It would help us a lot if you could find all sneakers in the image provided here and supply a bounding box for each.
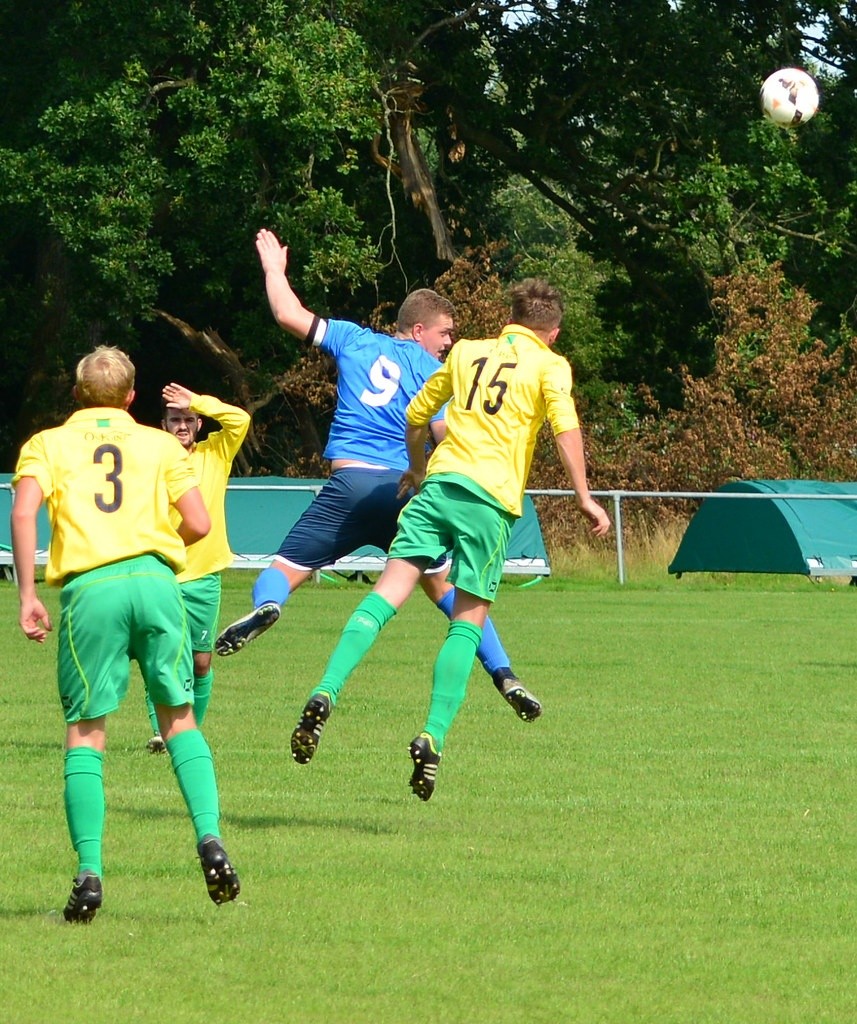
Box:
[195,837,241,905]
[146,732,170,759]
[290,692,333,764]
[500,678,544,723]
[407,733,441,801]
[214,602,281,656]
[63,867,102,925]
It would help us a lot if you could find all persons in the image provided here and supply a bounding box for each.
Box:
[145,383,250,754]
[290,276,610,802]
[10,345,241,923]
[213,228,542,723]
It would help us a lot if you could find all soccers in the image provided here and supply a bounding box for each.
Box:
[758,67,820,129]
